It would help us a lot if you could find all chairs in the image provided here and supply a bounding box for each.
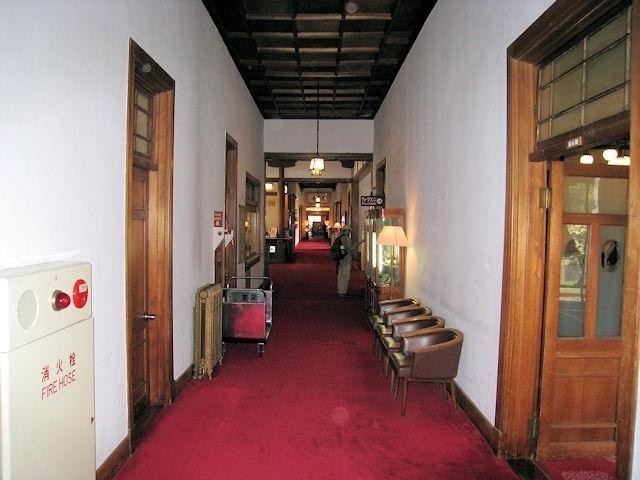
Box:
[369,298,463,417]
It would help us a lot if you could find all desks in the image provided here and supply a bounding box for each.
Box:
[264,235,283,264]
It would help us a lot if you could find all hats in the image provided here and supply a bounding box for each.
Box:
[343,226,352,231]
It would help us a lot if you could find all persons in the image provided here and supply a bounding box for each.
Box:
[334,224,355,298]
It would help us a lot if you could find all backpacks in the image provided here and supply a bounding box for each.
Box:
[332,234,347,261]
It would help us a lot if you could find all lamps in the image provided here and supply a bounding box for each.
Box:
[603,148,618,162]
[377,225,409,300]
[303,221,310,240]
[315,188,321,203]
[580,149,594,165]
[325,221,342,236]
[309,80,325,176]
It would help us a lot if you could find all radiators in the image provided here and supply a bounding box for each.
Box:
[193,284,223,380]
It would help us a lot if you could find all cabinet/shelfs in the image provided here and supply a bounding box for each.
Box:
[364,208,405,317]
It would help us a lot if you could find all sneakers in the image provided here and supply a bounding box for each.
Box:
[337,292,352,298]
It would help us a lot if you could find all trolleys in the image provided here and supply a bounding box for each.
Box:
[221,276,273,356]
